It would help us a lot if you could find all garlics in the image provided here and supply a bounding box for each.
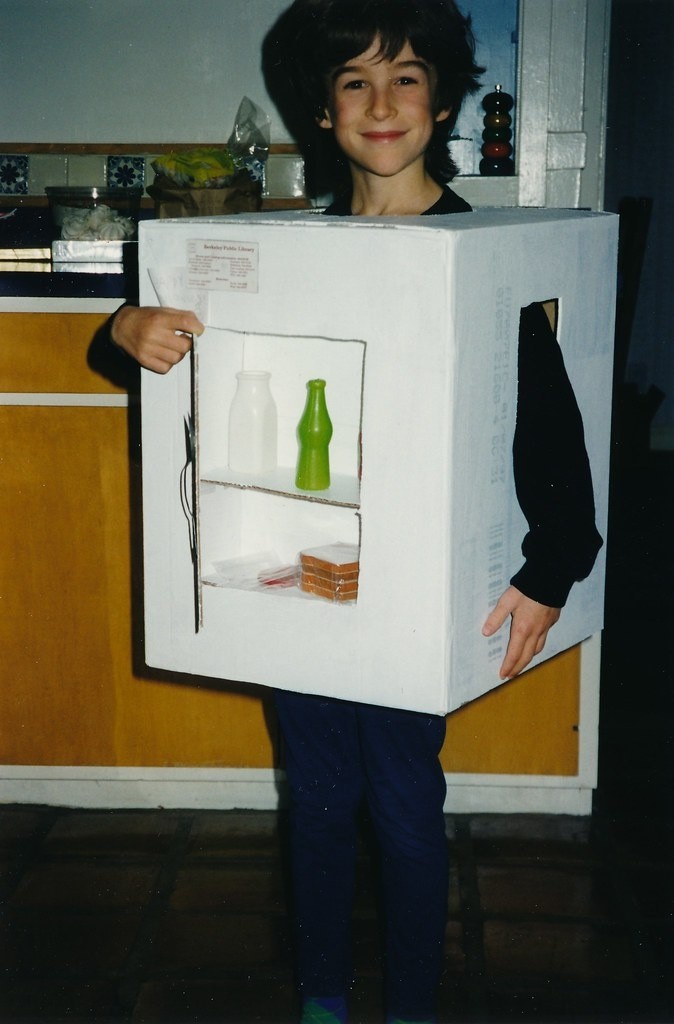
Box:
[61,206,135,241]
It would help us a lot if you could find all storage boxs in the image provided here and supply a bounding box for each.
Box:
[136,209,621,716]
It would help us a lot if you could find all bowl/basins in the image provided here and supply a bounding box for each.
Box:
[45,185,146,241]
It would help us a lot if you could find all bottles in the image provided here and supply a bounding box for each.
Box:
[227,372,277,479]
[297,379,335,491]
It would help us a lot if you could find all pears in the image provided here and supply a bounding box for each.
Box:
[156,149,235,187]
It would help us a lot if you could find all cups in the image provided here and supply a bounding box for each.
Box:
[448,139,477,176]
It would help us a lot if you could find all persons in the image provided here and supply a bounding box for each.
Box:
[110,0,603,1024]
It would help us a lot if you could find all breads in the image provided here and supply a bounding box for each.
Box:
[300,543,360,601]
